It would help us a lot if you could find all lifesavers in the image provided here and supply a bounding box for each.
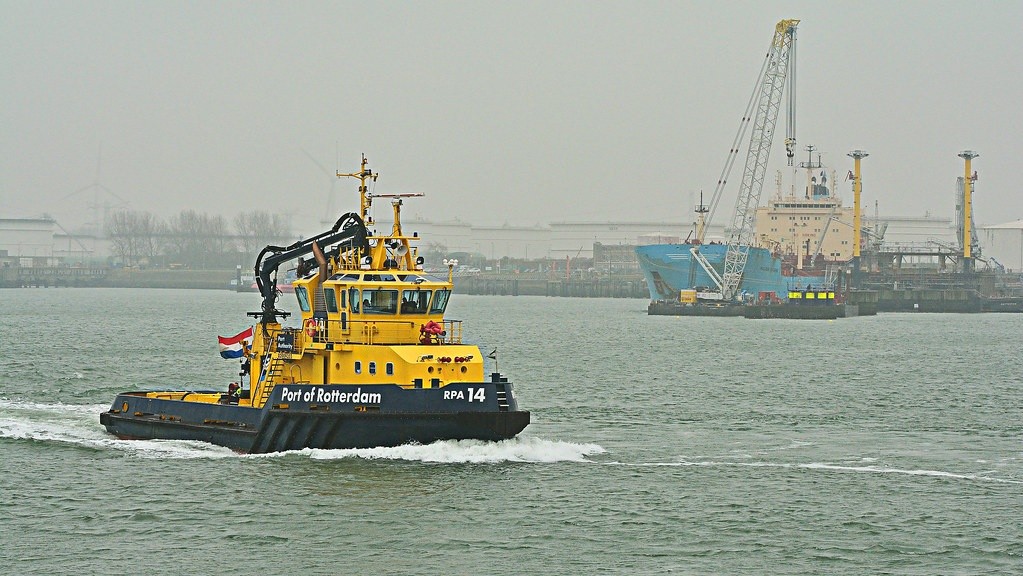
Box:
[307,319,316,337]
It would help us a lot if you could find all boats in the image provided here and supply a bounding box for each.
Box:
[634,167,870,300]
[98,151,534,452]
[229,264,315,295]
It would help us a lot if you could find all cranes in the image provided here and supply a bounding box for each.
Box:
[683,19,798,304]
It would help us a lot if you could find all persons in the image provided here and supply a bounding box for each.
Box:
[228,382,241,397]
[363,299,371,307]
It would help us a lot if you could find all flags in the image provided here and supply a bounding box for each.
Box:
[486,350,495,359]
[218,326,254,359]
